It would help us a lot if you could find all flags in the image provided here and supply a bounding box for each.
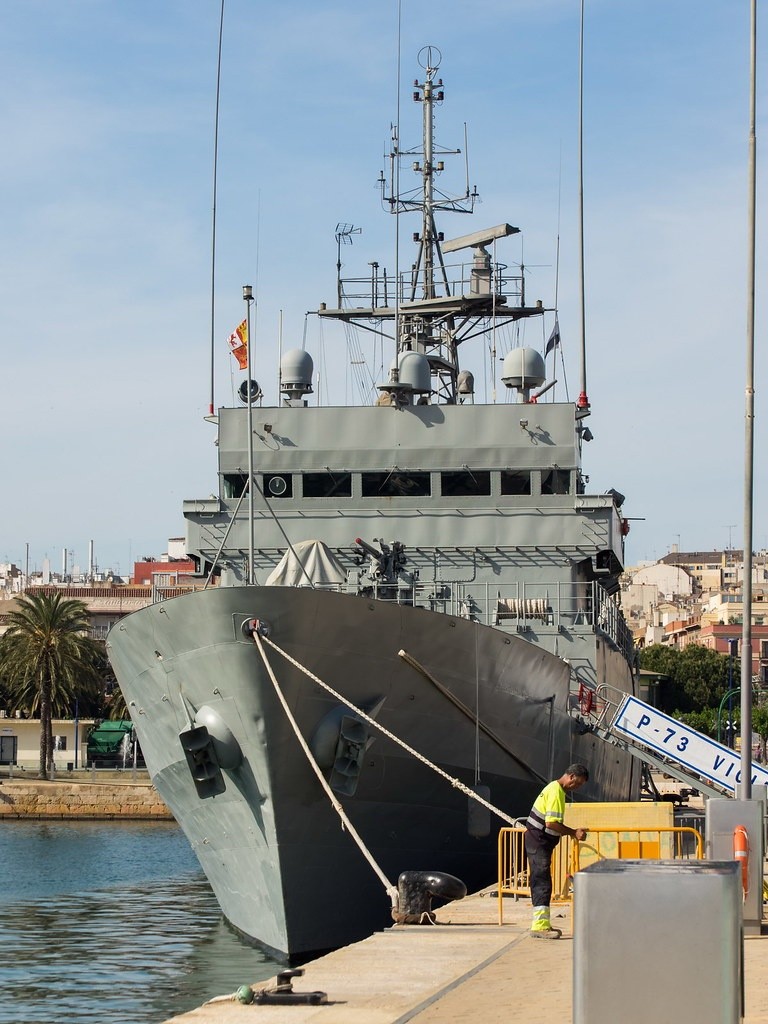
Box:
[226,318,248,369]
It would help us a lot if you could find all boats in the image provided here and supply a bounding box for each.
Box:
[106,0,656,965]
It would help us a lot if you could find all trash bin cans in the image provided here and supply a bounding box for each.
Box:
[66,763,73,770]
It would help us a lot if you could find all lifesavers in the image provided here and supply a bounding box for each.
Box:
[732,821,750,902]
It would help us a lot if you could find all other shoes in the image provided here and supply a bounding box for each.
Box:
[531,928,562,939]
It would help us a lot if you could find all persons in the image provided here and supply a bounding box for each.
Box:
[524,764,589,939]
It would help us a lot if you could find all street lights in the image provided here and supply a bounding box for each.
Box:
[725,636,739,749]
